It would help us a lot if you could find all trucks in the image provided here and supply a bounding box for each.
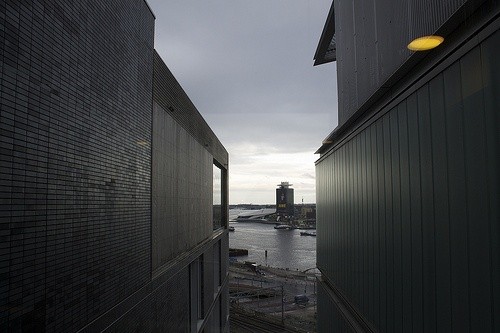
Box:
[245,261,261,272]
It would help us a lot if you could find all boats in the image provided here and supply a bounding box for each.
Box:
[300,231,316,237]
[229,225,235,231]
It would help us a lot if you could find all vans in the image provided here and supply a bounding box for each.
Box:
[295,294,309,304]
